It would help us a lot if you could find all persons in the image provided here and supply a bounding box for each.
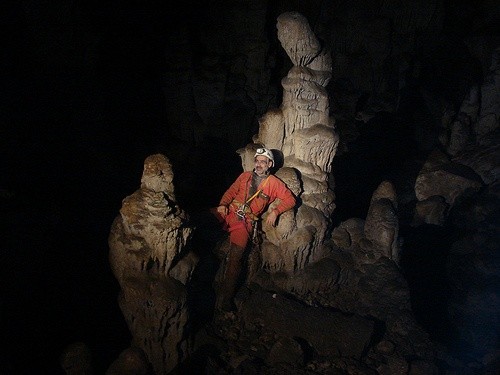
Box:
[188,148,296,314]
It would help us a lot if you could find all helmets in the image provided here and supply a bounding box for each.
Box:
[254,148,274,168]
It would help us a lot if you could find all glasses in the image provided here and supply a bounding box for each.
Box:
[254,160,268,164]
